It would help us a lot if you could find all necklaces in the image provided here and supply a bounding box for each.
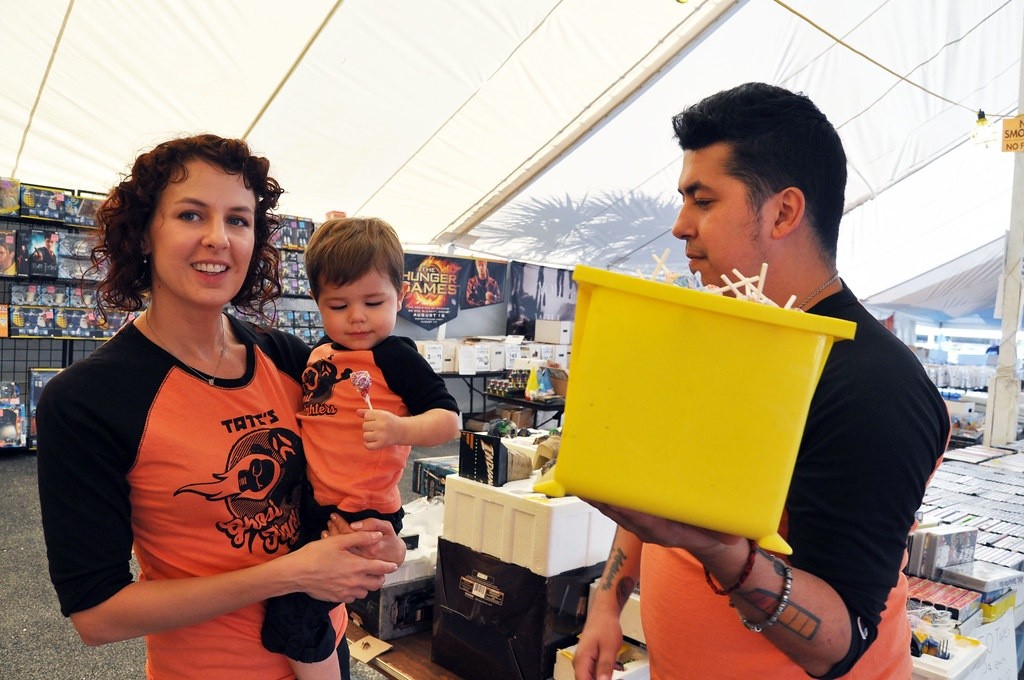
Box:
[797,272,838,311]
[146,308,225,386]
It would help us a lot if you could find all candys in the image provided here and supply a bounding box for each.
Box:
[349,370,373,409]
[633,248,807,313]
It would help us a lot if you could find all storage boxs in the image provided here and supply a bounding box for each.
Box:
[533,265,858,555]
[443,473,617,577]
[412,456,459,497]
[431,535,606,680]
[414,319,573,375]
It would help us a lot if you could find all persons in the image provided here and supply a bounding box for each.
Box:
[466,260,501,306]
[0,240,16,276]
[35,135,407,680]
[261,218,460,679]
[573,82,955,680]
[31,234,57,274]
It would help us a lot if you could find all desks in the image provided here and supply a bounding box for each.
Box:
[436,369,513,412]
[482,393,566,429]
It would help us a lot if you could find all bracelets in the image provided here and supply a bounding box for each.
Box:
[742,558,790,632]
[703,542,755,595]
